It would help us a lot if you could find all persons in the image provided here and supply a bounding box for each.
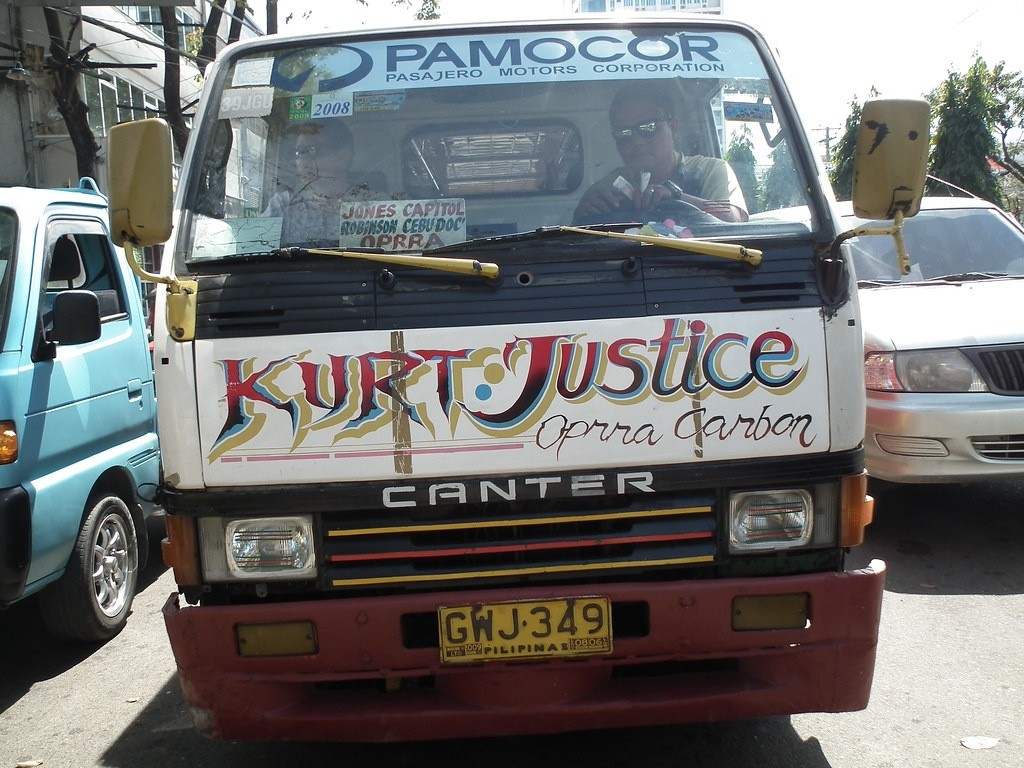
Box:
[259,117,392,243]
[571,87,750,227]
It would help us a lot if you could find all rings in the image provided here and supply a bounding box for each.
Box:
[649,188,655,192]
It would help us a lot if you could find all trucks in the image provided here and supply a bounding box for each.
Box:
[102,4,939,752]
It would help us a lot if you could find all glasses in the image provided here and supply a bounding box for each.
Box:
[613,115,673,146]
[294,143,342,161]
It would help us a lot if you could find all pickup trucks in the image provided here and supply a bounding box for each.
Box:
[0,179,178,644]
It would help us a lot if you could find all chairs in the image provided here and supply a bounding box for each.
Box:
[47,238,81,288]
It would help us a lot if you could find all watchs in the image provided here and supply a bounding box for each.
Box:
[662,180,683,200]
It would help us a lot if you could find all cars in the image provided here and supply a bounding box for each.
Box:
[738,198,1023,496]
